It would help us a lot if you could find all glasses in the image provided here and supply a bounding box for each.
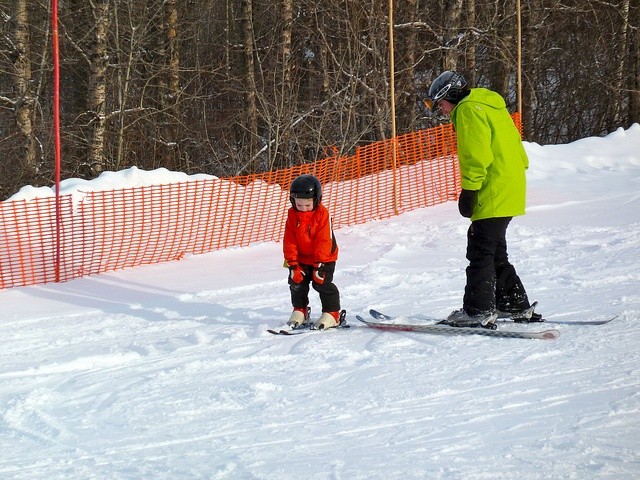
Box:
[422,84,452,113]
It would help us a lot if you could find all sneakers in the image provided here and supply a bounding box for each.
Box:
[494,307,530,318]
[315,308,341,329]
[287,308,308,328]
[448,307,497,325]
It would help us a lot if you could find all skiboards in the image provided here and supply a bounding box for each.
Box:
[356,310,619,339]
[267,311,350,335]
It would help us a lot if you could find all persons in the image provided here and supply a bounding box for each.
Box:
[282,174,341,330]
[427,70,531,324]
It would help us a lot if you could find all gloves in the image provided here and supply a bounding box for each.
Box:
[458,189,479,218]
[287,261,306,284]
[312,262,326,284]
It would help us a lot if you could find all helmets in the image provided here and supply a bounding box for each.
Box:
[424,70,468,113]
[290,174,322,210]
